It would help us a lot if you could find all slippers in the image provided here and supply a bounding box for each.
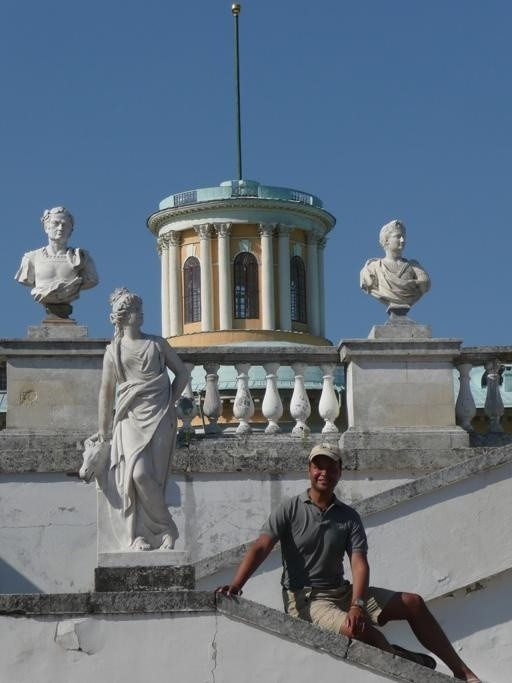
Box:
[391,644,437,670]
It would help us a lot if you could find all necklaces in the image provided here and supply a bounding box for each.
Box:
[309,495,335,511]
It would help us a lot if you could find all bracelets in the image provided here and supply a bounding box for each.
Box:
[350,598,369,610]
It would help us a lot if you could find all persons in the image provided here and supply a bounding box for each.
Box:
[357,218,431,310]
[94,283,188,553]
[14,206,99,306]
[216,443,480,683]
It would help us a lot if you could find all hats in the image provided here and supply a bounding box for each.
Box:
[307,442,344,463]
